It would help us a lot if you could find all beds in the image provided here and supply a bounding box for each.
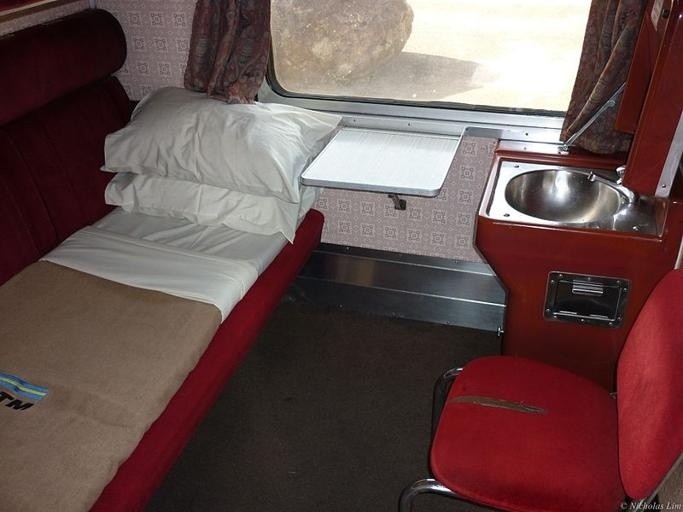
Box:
[0,8,323,512]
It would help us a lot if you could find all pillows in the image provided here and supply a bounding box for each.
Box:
[100,87,344,245]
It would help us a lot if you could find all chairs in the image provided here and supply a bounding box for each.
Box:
[398,269,683,512]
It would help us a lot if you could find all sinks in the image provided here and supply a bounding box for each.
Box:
[505,170,634,224]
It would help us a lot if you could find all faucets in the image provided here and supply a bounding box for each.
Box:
[616,165,626,185]
